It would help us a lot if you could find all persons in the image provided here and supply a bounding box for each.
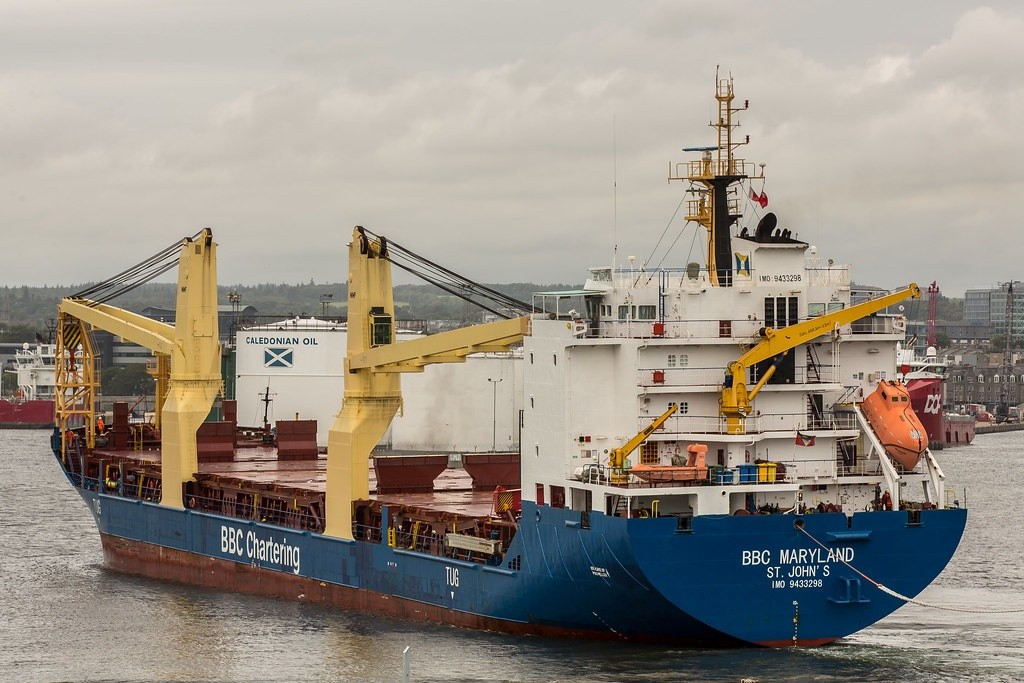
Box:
[875,486,893,511]
[66,428,75,448]
[97,417,105,434]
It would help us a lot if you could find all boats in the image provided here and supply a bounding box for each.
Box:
[0,332,90,428]
[899,372,976,450]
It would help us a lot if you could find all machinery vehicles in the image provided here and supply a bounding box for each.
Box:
[996,281,1021,425]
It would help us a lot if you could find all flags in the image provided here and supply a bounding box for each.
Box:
[749,186,768,209]
[795,431,816,446]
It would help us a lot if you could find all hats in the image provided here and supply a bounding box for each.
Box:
[99,417,102,419]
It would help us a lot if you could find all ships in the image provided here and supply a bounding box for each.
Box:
[49,66,969,652]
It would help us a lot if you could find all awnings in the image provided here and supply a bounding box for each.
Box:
[530,289,605,321]
